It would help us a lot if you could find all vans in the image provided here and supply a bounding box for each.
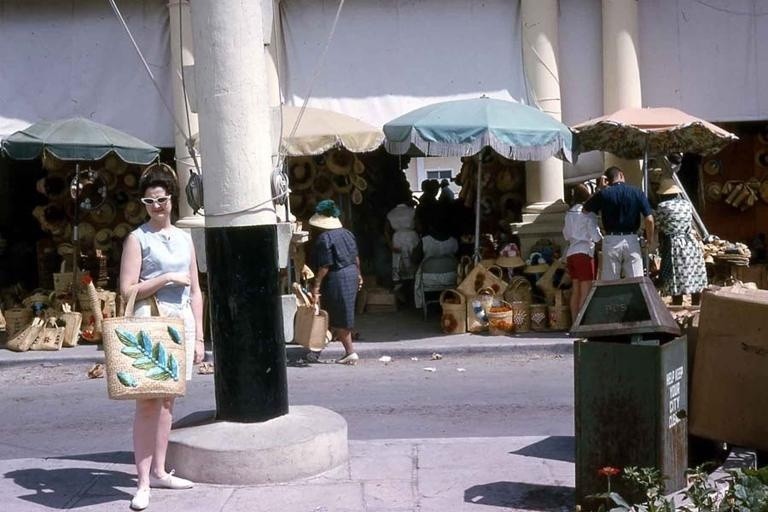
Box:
[336,351,359,365]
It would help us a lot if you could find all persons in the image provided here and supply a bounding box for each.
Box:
[423,179,440,207]
[581,165,655,281]
[654,178,710,306]
[309,199,363,364]
[117,165,206,510]
[418,180,429,204]
[439,179,453,203]
[410,214,459,311]
[561,184,601,326]
[383,195,422,284]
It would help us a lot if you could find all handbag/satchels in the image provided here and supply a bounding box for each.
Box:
[314,277,320,289]
[196,335,205,343]
[594,187,601,193]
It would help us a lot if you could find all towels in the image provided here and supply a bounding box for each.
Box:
[569,278,688,512]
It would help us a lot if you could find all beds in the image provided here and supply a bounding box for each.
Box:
[572,104,740,198]
[189,102,384,218]
[1,117,164,313]
[382,94,573,264]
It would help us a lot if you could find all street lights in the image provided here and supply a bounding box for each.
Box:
[294,296,328,352]
[439,265,569,335]
[1,259,119,353]
[100,282,190,401]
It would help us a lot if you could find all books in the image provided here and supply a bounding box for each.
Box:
[691,285,767,455]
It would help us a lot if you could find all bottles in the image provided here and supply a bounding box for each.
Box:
[494,243,550,275]
[307,199,344,229]
[285,149,357,219]
[32,156,147,259]
[455,146,525,222]
[655,177,684,195]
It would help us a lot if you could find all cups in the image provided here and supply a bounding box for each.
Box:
[141,195,173,205]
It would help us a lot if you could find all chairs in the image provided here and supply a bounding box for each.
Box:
[130,485,150,511]
[149,471,197,490]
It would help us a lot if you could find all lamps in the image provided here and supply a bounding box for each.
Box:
[419,252,463,320]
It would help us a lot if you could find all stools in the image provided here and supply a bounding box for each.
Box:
[604,230,639,236]
